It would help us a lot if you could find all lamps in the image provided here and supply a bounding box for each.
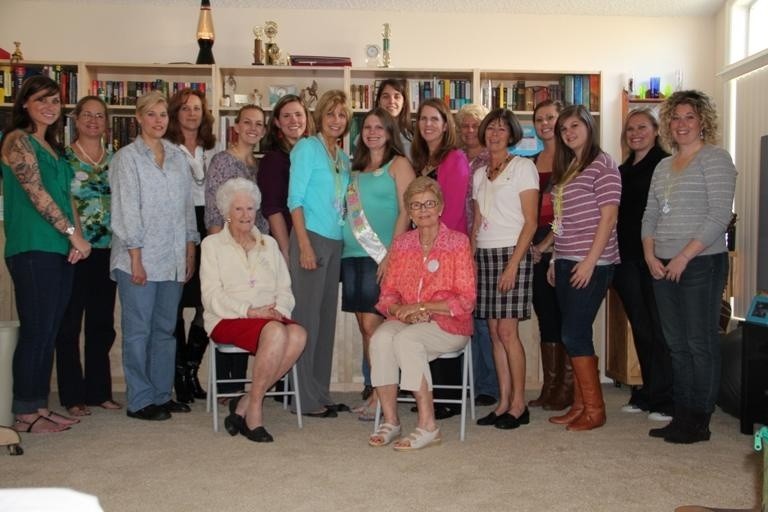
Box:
[196,0,217,63]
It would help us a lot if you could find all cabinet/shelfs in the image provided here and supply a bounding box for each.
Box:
[78,63,215,393]
[474,71,604,391]
[605,85,733,394]
[0,60,78,393]
[215,68,347,392]
[349,70,474,391]
[738,319,767,435]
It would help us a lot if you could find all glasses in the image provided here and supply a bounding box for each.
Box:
[77,110,106,122]
[410,199,437,210]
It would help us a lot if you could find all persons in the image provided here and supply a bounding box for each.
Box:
[1,64,207,162]
[2,75,575,433]
[198,174,307,443]
[613,106,679,424]
[637,87,739,443]
[544,103,624,431]
[368,173,480,450]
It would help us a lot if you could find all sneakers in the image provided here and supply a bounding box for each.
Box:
[622,403,649,413]
[663,424,710,443]
[648,413,674,420]
[362,385,372,400]
[397,387,407,403]
[648,422,677,437]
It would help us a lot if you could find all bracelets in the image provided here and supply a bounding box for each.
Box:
[418,302,426,309]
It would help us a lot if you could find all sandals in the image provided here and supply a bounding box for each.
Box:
[368,423,400,448]
[47,411,80,424]
[393,426,441,451]
[15,415,71,432]
[217,397,231,405]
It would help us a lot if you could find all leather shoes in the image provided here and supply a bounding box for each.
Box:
[324,403,350,412]
[240,420,273,443]
[411,406,418,412]
[495,406,530,430]
[158,399,191,413]
[434,407,461,419]
[477,410,510,425]
[291,407,337,419]
[126,404,172,421]
[224,395,245,437]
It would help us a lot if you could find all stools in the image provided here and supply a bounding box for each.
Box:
[205,338,304,430]
[373,336,476,444]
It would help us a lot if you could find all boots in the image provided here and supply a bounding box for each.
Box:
[186,323,209,400]
[528,342,562,407]
[549,367,585,425]
[542,343,574,411]
[174,318,196,404]
[566,356,607,431]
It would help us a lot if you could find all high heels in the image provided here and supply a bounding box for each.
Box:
[86,399,121,410]
[67,402,91,416]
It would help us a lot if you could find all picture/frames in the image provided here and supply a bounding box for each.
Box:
[744,295,768,324]
